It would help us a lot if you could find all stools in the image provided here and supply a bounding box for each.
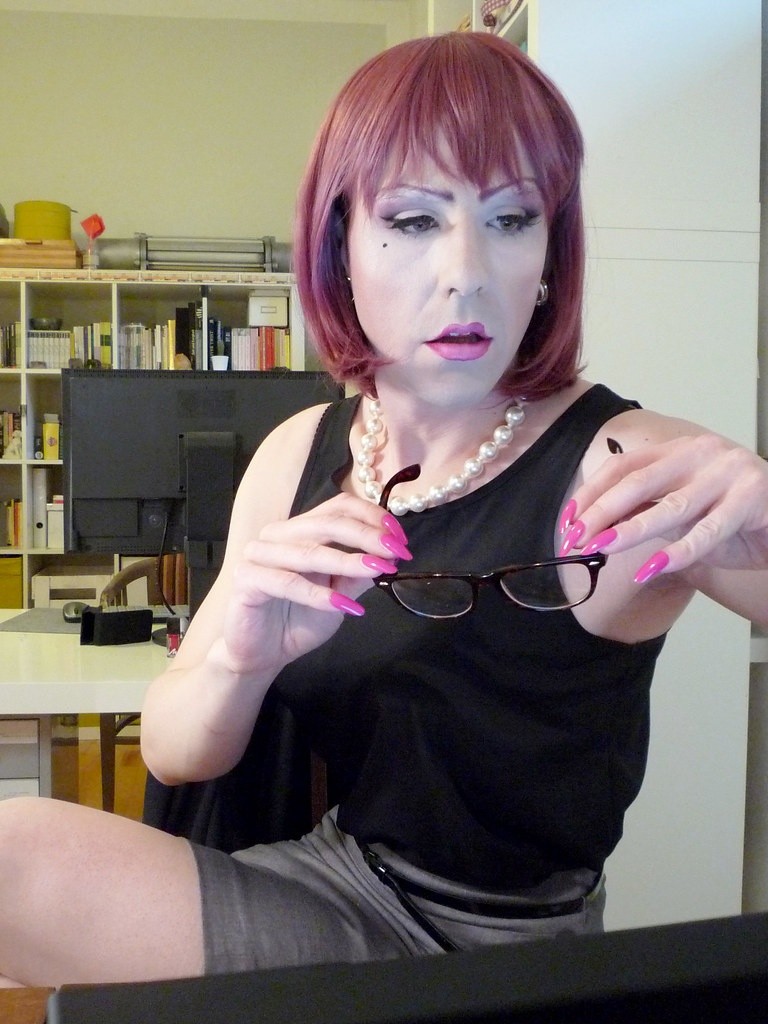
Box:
[32,565,115,606]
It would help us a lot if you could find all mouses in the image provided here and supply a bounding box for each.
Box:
[63,602,87,624]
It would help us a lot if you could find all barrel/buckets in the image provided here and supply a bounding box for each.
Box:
[14,200,77,239]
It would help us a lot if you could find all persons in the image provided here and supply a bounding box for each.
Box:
[0,34,768,989]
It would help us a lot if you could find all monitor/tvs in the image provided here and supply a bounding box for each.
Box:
[59,369,345,620]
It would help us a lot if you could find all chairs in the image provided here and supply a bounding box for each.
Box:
[102,556,187,816]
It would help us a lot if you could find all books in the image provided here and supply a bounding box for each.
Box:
[0,300,292,549]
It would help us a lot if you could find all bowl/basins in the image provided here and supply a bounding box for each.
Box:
[29,319,62,329]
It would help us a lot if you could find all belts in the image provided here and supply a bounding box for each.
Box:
[348,838,606,952]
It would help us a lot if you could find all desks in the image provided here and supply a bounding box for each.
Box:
[1,609,178,801]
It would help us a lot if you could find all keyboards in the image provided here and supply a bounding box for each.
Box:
[103,605,190,623]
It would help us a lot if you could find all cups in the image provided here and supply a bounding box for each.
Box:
[211,355,229,371]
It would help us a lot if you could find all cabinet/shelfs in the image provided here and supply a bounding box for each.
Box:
[428,0,760,933]
[0,268,307,744]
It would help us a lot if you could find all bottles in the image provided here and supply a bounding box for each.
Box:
[83,233,100,281]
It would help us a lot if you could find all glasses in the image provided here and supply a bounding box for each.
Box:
[376,436,626,621]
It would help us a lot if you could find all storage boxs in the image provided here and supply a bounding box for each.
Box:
[247,296,288,328]
[47,504,64,547]
[1,238,82,269]
[43,422,64,460]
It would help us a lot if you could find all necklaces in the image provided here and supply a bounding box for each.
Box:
[357,392,526,517]
